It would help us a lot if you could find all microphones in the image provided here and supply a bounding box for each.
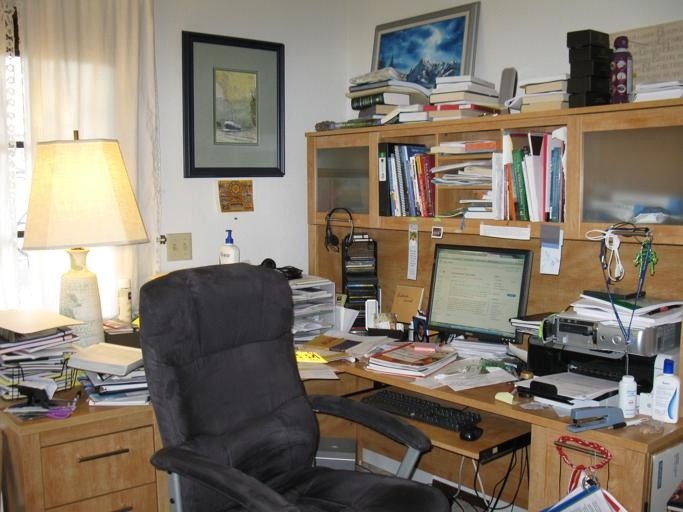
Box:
[345,247,351,262]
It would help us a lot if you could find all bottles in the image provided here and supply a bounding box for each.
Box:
[118,278,132,323]
[609,36,633,104]
[620,374,637,420]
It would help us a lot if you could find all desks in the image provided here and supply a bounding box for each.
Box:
[1,348,682,512]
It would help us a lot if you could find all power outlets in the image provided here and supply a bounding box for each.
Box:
[165,233,193,261]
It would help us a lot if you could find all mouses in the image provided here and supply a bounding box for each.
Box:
[460,426,483,441]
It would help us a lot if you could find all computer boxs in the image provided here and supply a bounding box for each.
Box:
[541,309,681,357]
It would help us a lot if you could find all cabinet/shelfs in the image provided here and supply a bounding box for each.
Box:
[304,100,682,347]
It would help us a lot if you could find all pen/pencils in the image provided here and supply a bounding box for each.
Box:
[608,418,642,430]
[70,390,81,411]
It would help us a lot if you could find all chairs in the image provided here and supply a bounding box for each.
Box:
[138,261,449,511]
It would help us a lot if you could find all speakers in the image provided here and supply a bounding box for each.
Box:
[365,299,378,331]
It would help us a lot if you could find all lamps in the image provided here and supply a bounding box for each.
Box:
[22,130,151,346]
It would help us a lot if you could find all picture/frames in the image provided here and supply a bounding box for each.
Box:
[181,30,287,178]
[369,1,479,91]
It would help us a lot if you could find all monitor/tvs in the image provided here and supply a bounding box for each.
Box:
[425,243,533,344]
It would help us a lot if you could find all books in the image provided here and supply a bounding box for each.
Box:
[375,126,569,226]
[633,80,682,102]
[288,276,458,386]
[0,304,152,429]
[505,73,570,113]
[508,289,683,337]
[326,66,508,130]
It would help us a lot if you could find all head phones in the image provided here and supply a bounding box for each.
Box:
[325,207,354,247]
[261,258,301,278]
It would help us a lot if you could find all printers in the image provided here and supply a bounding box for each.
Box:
[517,335,657,410]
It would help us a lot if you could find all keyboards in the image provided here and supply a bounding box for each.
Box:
[361,389,481,432]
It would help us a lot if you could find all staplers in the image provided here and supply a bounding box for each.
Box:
[566,406,624,433]
